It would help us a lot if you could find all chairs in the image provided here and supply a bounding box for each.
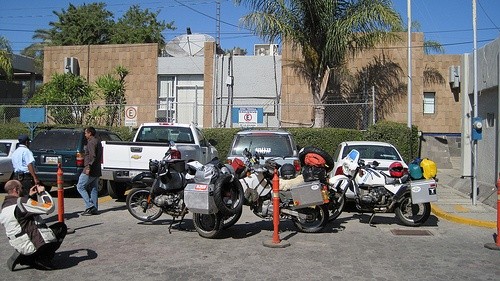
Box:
[142,133,157,142]
[176,132,189,142]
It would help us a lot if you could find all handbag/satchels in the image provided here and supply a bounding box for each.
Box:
[194,157,223,185]
[408,155,437,179]
[303,153,328,182]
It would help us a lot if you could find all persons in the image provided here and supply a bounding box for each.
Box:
[2,179,67,272]
[11,134,40,203]
[77,127,102,216]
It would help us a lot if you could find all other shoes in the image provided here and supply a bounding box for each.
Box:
[35,258,54,270]
[91,212,97,215]
[8,252,19,271]
[83,207,96,215]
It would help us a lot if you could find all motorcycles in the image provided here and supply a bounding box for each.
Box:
[219,140,337,234]
[125,140,244,238]
[315,148,439,227]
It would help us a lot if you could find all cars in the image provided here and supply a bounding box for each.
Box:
[326,140,409,213]
[0,139,29,193]
[25,126,124,197]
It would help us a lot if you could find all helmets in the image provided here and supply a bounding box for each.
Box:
[280,164,296,178]
[389,162,404,177]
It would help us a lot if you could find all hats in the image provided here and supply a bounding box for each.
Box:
[18,135,31,141]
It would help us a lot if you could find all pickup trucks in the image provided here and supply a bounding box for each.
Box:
[99,122,219,201]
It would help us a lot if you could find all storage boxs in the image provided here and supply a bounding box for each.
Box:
[411,179,437,205]
[290,181,326,206]
[183,183,219,215]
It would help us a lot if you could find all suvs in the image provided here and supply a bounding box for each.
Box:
[225,128,302,178]
